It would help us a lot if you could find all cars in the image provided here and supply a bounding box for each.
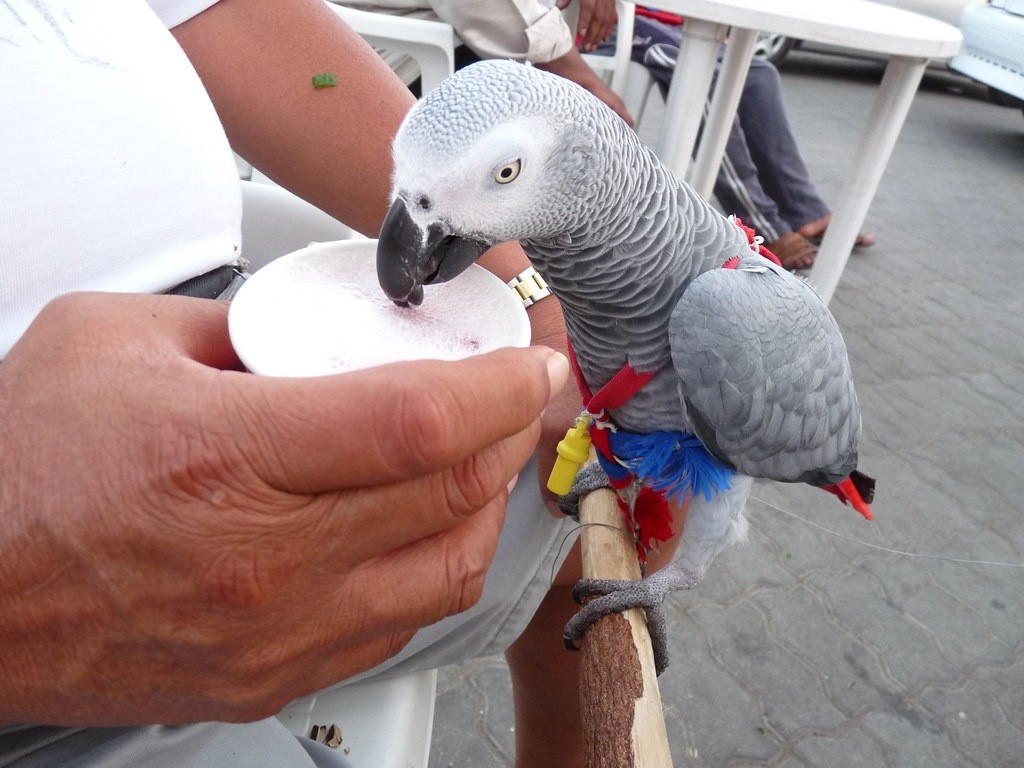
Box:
[717,0,993,95]
[953,0,1023,102]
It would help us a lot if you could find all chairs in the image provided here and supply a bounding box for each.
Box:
[251,0,454,185]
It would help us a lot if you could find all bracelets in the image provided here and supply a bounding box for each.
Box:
[507,266,554,308]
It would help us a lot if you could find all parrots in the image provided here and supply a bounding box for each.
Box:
[374,56,877,679]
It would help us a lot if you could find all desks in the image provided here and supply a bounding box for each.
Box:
[609,0,961,306]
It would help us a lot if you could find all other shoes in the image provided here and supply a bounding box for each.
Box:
[762,212,877,269]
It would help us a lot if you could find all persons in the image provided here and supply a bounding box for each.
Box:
[0,0,584,768]
[326,0,634,130]
[577,0,877,270]
